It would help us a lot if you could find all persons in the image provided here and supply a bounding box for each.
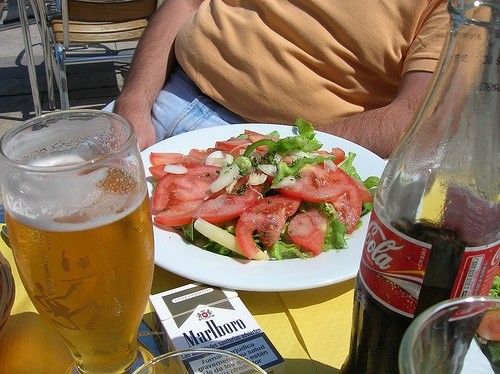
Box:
[78,0,469,160]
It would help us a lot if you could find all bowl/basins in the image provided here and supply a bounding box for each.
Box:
[0,251,16,341]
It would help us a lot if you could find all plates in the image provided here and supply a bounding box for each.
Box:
[140,122,388,292]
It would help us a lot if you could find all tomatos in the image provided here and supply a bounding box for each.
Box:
[145,128,372,260]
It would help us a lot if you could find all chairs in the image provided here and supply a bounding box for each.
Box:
[0,0,166,132]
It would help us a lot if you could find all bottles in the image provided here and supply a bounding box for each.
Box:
[335,0,500,374]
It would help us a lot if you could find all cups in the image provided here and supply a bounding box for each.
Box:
[131,348,267,374]
[398,296,500,374]
[0,109,155,374]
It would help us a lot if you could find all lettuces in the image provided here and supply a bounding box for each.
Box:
[181,117,380,262]
